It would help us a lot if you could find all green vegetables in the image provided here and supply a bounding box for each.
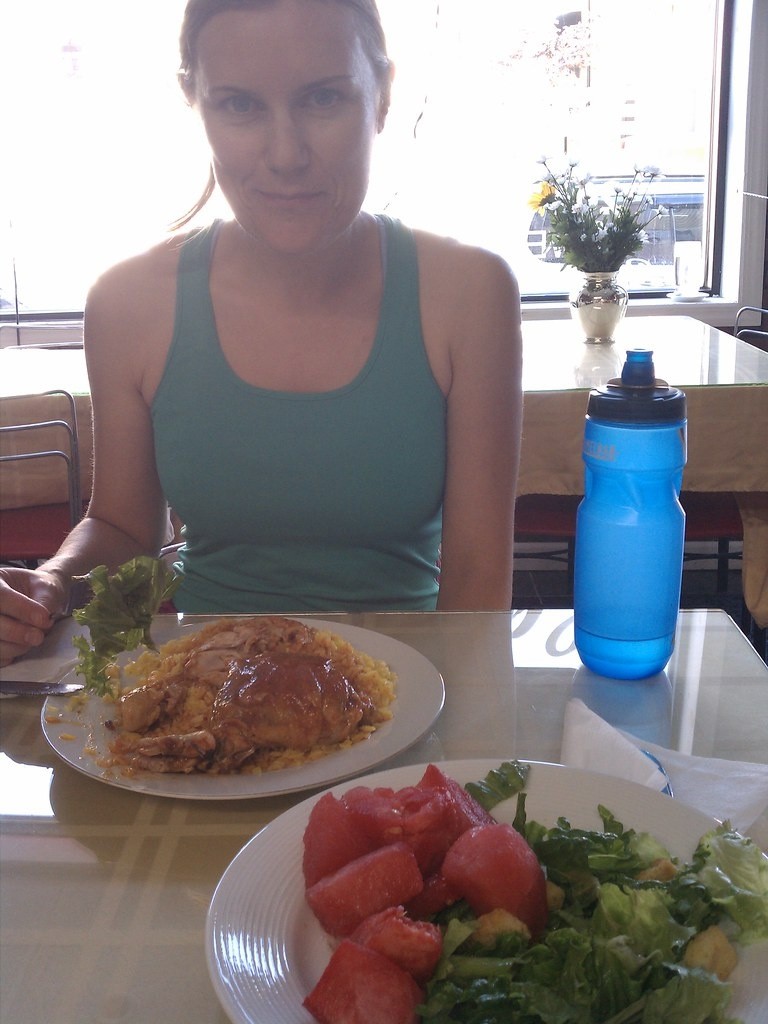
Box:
[68,556,184,699]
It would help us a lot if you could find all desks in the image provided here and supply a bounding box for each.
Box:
[0,313,768,589]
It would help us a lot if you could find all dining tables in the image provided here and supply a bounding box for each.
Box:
[0,605,768,1024]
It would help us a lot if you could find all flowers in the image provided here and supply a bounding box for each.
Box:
[524,152,669,275]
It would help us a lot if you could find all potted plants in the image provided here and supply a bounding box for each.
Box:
[568,272,629,345]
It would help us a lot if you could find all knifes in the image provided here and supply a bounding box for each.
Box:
[0,681,86,696]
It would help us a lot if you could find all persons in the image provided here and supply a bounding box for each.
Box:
[0,0,523,668]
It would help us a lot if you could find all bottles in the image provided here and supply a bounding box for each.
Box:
[575,349,689,679]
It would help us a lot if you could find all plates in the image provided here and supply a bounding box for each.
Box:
[40,616,445,799]
[205,758,768,1024]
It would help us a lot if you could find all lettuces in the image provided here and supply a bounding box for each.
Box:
[400,761,768,1024]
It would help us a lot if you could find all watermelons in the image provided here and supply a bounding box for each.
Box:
[299,761,547,1024]
